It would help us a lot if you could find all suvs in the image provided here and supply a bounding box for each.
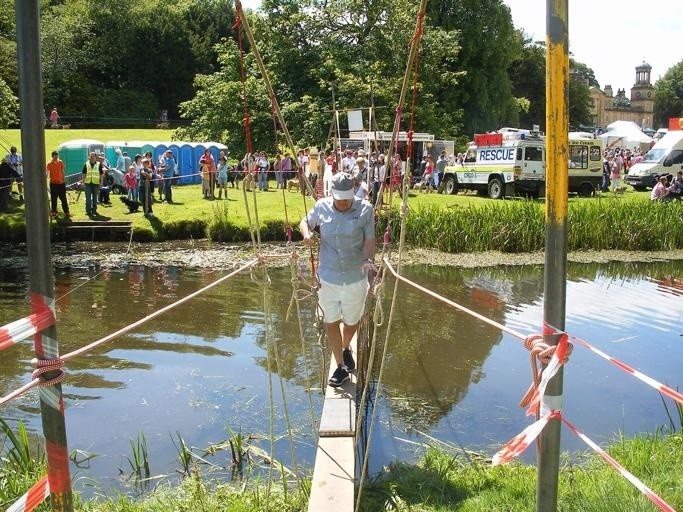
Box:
[328,125,608,202]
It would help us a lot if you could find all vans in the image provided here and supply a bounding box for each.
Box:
[620,127,682,193]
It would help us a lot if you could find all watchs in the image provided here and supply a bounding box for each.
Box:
[362,258,375,264]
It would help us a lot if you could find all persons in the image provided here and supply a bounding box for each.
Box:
[43,109,48,129]
[300,172,378,385]
[156,110,167,128]
[650,165,683,203]
[2,146,178,216]
[601,147,647,193]
[420,150,467,194]
[50,107,60,126]
[199,148,311,201]
[315,149,402,206]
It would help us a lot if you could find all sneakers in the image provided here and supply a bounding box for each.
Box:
[328,364,349,387]
[342,347,354,373]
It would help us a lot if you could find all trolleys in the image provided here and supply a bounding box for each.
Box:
[106,168,127,194]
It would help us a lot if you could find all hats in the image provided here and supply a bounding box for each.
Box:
[329,172,354,201]
